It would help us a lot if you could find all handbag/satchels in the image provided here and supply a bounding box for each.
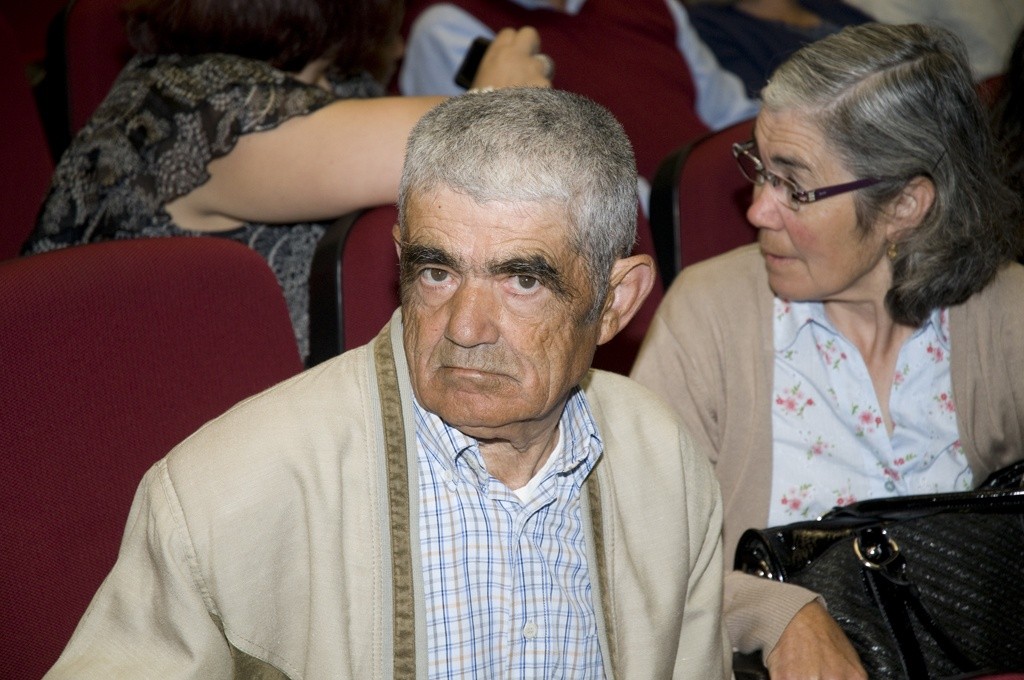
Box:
[732,459,1024,679]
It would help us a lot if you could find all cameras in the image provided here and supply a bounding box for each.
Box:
[454,36,492,90]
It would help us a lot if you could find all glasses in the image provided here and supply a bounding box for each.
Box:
[732,141,931,204]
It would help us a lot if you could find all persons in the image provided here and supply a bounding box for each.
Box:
[24,0,556,368]
[631,22,1024,680]
[43,88,732,680]
[665,0,1024,135]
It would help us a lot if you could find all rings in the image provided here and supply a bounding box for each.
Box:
[535,54,554,77]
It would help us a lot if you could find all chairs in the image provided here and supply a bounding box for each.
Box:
[0,0,762,680]
